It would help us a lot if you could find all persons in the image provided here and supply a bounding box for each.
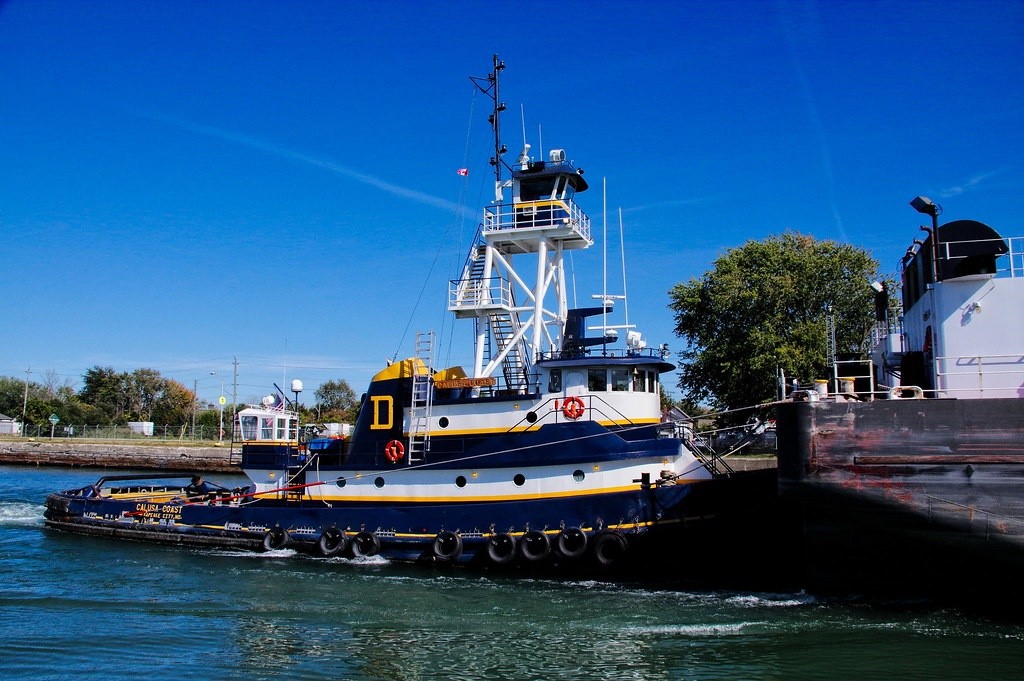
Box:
[186,476,210,498]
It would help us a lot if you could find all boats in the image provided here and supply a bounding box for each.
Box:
[39,50,1024,609]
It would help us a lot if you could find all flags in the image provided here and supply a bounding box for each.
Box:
[456,167,469,177]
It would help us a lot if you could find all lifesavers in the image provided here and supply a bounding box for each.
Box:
[386,440,405,461]
[563,396,585,418]
